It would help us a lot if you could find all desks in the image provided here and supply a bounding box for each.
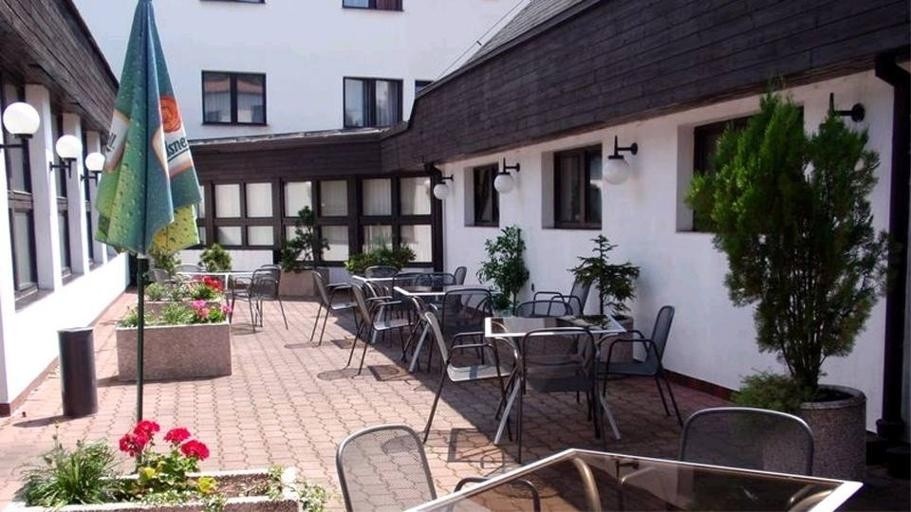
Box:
[413,448,865,512]
[483,312,629,445]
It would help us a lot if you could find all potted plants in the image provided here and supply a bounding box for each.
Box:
[687,84,880,480]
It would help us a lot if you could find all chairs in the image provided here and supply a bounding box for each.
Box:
[595,304,680,418]
[421,310,517,446]
[514,326,607,465]
[312,264,598,382]
[153,261,286,333]
[331,423,541,512]
[679,409,817,495]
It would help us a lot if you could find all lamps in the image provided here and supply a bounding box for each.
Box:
[0,95,39,152]
[84,151,105,185]
[434,174,455,200]
[43,130,81,172]
[493,157,521,195]
[603,136,638,184]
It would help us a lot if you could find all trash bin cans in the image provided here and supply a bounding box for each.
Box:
[57,327,98,416]
[129,254,149,286]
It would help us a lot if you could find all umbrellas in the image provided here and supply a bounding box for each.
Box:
[91,0,204,474]
